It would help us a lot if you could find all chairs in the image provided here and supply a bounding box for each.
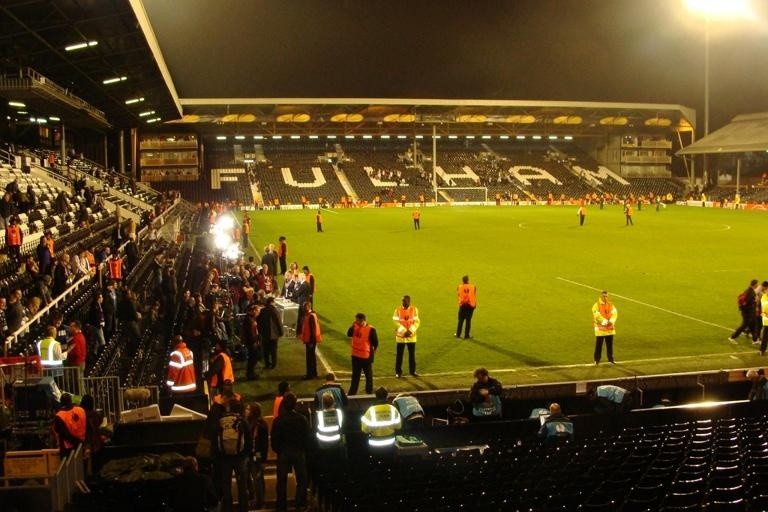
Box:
[0,133,215,427]
[690,184,768,211]
[215,128,685,210]
[303,381,766,512]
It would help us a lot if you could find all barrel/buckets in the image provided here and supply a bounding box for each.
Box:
[12,374,56,449]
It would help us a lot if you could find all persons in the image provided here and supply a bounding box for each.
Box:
[0,137,768,512]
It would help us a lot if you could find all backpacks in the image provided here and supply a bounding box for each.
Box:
[215,414,247,458]
[737,293,748,310]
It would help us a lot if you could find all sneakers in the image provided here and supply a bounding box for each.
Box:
[760,349,765,355]
[453,333,460,338]
[727,337,739,346]
[303,372,318,380]
[464,335,474,339]
[395,369,402,378]
[751,339,763,345]
[409,370,419,377]
[594,361,600,368]
[608,360,617,365]
